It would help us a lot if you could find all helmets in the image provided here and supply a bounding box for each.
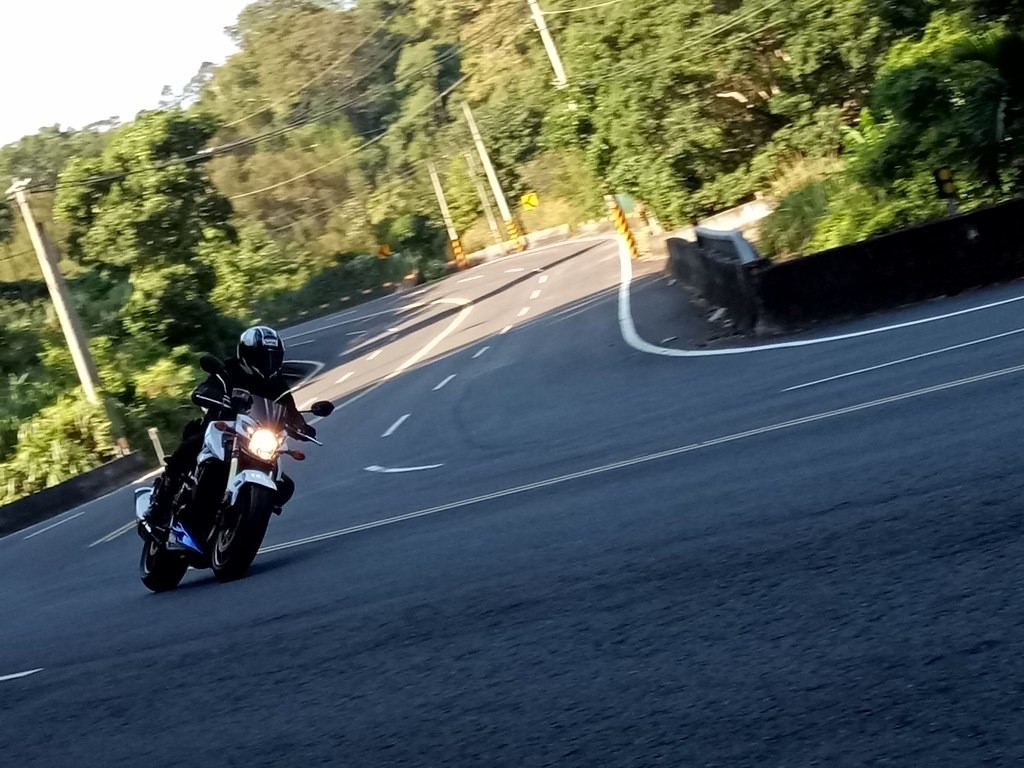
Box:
[237,325,285,380]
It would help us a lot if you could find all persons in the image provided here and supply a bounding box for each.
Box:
[143,326,316,528]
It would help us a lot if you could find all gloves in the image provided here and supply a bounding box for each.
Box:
[297,425,317,442]
[200,388,221,406]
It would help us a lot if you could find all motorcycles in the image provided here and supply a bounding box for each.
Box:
[133,356,334,595]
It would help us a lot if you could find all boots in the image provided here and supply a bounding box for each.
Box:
[144,478,175,526]
